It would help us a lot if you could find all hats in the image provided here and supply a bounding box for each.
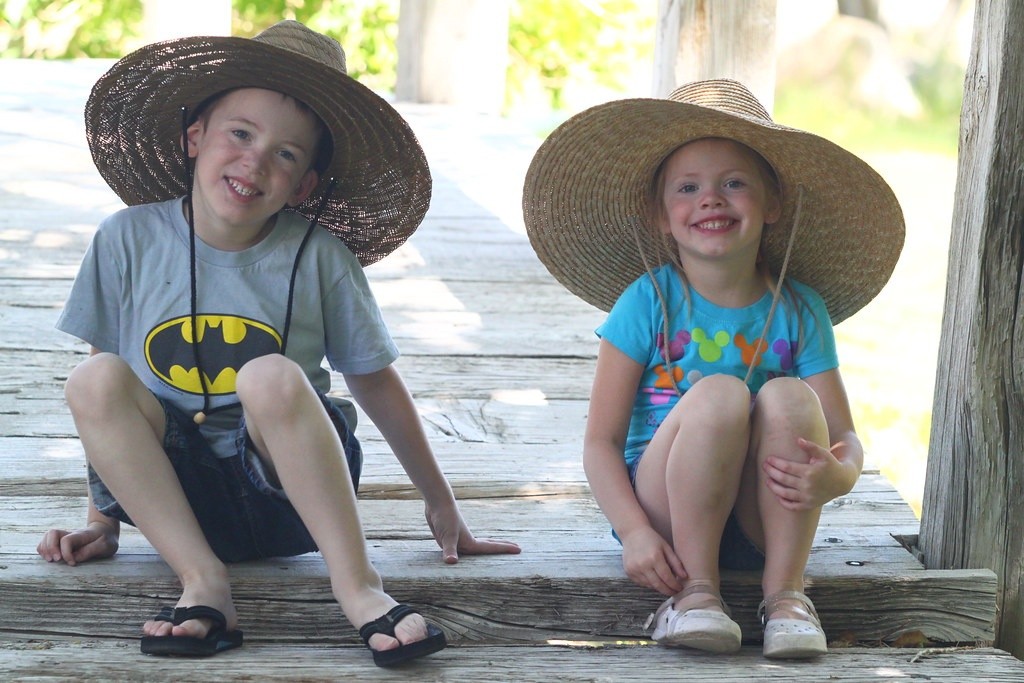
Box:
[83,21,431,268]
[521,80,907,323]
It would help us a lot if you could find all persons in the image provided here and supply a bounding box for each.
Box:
[36,19,519,666]
[523,78,906,658]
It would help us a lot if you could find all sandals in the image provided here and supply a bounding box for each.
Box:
[758,590,828,658]
[644,584,741,651]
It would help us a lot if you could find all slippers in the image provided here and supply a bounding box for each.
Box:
[141,604,243,658]
[359,601,446,665]
